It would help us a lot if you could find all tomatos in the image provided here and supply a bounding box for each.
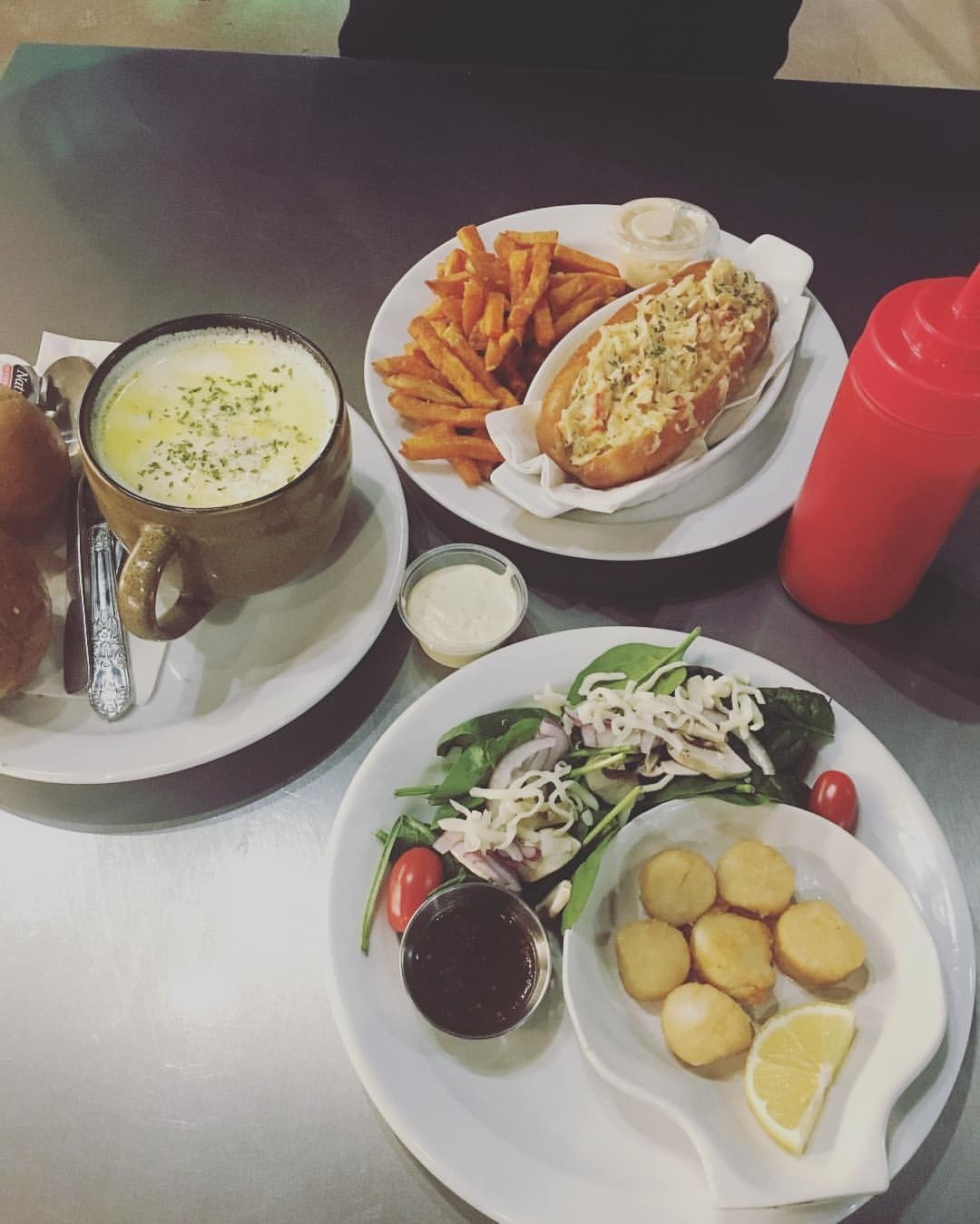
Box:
[386,846,442,932]
[809,770,856,831]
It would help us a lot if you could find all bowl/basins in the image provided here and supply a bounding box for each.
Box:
[398,881,552,1041]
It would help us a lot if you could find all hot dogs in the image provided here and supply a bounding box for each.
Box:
[535,256,777,487]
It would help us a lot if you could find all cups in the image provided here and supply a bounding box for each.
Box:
[75,311,353,640]
[605,195,720,289]
[394,542,529,668]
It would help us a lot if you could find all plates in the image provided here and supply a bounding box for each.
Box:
[0,404,408,785]
[363,201,849,562]
[560,794,948,1209]
[322,626,977,1224]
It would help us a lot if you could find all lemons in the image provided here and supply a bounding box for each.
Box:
[744,1002,857,1159]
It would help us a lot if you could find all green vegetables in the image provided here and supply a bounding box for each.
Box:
[361,626,836,948]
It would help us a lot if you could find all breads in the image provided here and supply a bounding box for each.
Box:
[0,384,70,708]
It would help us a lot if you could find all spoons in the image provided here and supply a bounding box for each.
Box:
[39,355,98,692]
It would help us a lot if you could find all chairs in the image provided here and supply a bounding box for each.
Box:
[337,0,817,78]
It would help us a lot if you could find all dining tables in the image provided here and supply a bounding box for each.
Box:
[0,45,980,1224]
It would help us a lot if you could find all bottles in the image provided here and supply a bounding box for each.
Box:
[777,260,980,621]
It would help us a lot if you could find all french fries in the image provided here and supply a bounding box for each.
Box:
[371,225,630,484]
